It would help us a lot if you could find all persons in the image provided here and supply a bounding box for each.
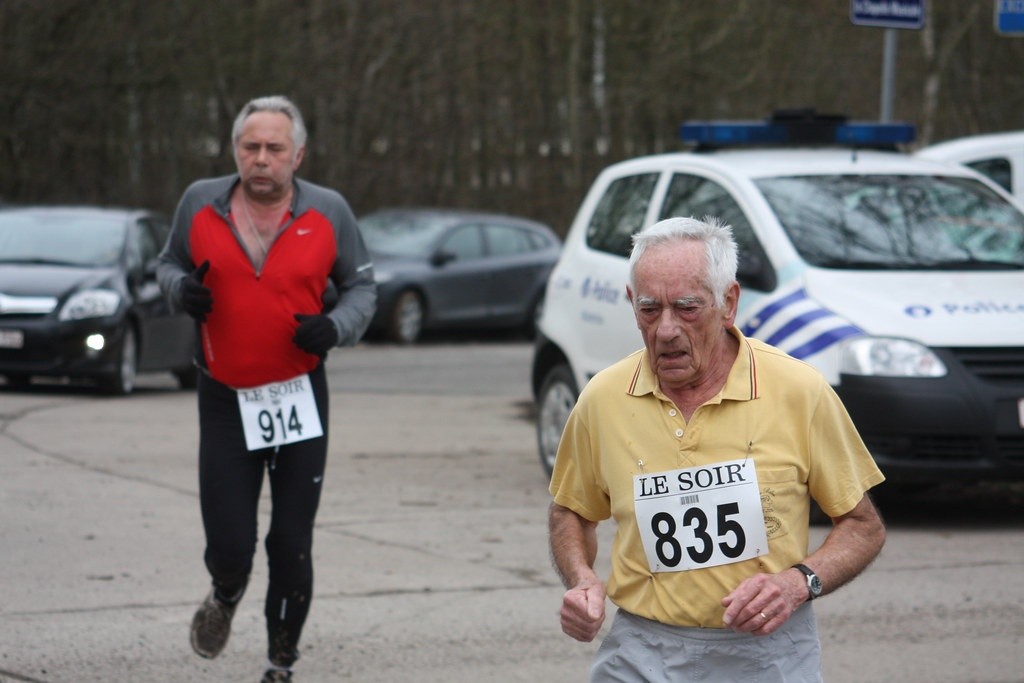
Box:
[547,216,889,683]
[155,97,379,683]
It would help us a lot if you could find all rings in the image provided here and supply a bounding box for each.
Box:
[759,612,769,622]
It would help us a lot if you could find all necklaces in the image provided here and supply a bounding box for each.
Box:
[239,187,269,255]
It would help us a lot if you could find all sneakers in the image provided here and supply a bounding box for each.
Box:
[259,668,293,683]
[190,588,237,660]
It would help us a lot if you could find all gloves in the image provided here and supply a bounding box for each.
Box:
[292,312,337,355]
[177,259,215,324]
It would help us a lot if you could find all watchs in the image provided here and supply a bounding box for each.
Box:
[791,564,823,602]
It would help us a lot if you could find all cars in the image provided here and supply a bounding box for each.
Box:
[910,131,1023,213]
[0,203,198,398]
[527,105,1024,526]
[352,206,561,346]
